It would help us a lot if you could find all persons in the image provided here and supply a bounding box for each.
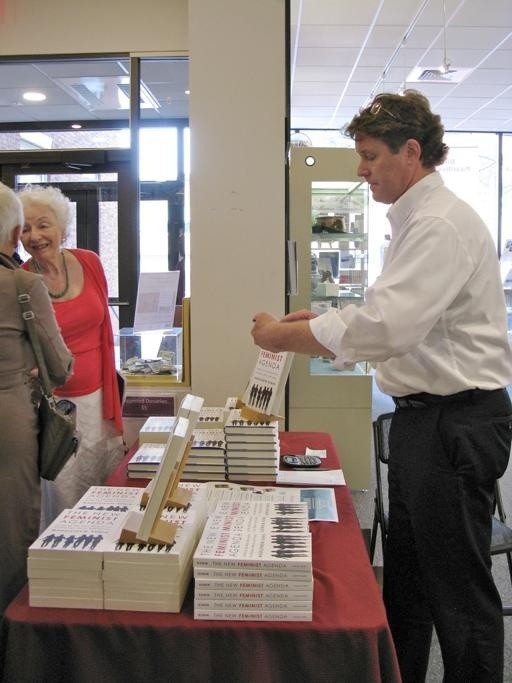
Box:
[248,87,509,681]
[0,178,77,619]
[15,184,127,535]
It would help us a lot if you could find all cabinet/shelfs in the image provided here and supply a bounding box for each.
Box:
[288,147,378,491]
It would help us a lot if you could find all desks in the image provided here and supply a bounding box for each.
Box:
[0,426,406,682]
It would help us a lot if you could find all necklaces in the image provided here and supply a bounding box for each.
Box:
[32,250,70,299]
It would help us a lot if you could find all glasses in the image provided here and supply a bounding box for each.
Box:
[369,101,399,121]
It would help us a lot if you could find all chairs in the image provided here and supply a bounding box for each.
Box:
[365,410,512,616]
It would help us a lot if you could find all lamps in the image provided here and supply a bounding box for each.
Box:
[398,39,412,97]
[437,0,457,72]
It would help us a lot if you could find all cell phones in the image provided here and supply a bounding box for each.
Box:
[283,455,321,468]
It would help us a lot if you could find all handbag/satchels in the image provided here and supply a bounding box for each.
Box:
[12,266,83,482]
